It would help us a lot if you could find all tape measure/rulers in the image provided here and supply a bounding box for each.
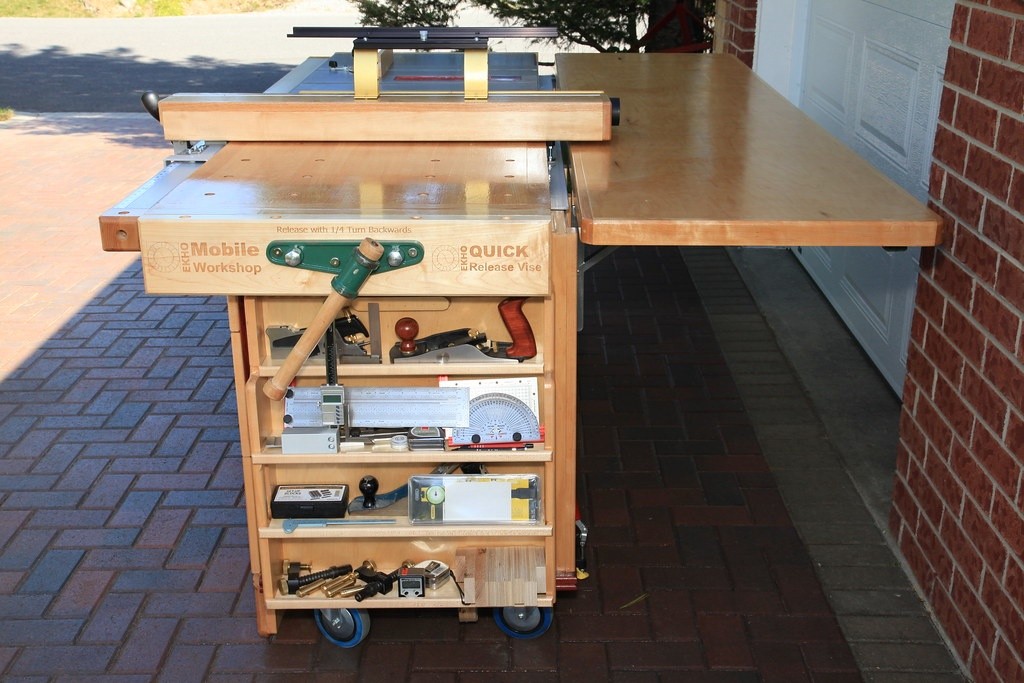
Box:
[282,517,398,534]
[412,559,451,590]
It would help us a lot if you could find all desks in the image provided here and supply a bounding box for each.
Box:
[555,52,946,251]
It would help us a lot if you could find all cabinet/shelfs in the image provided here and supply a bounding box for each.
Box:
[246,294,557,610]
